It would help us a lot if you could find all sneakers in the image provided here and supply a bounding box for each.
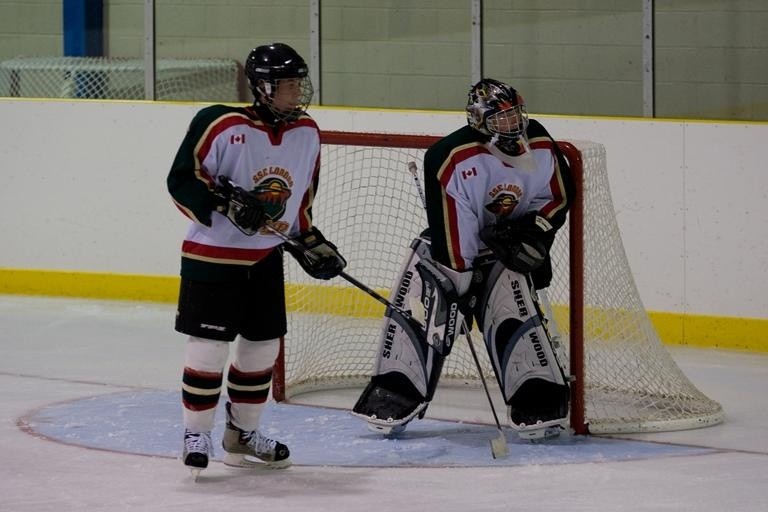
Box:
[222,422,289,462]
[183,430,211,468]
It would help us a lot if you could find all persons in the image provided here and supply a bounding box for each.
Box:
[168,43,346,467]
[353,78,572,443]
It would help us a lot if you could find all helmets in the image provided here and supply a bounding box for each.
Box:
[245,43,308,80]
[465,79,527,136]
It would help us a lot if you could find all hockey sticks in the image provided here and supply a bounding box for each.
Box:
[214,183,426,330]
[408,162,507,459]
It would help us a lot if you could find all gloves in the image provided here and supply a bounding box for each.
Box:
[215,185,266,236]
[479,210,558,274]
[283,226,347,280]
